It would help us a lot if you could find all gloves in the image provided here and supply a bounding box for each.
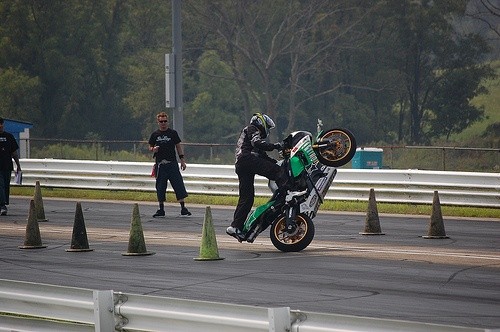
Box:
[274,143,283,150]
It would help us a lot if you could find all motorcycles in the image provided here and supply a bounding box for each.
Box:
[243,127,359,252]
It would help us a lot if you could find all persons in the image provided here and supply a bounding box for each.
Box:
[148,111,193,217]
[226,111,306,240]
[0,117,22,215]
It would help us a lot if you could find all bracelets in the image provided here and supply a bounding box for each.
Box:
[152,147,154,151]
[178,154,184,159]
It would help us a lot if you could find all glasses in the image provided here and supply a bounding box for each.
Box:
[159,120,167,123]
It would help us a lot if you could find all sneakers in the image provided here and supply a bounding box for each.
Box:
[285,188,308,205]
[227,226,246,239]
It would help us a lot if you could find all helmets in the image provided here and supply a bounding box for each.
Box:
[250,113,275,139]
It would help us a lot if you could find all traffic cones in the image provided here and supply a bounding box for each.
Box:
[192,206,226,260]
[358,189,387,236]
[120,203,156,257]
[25,180,49,222]
[421,189,450,240]
[17,199,48,249]
[64,202,93,252]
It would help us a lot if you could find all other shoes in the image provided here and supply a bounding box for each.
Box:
[181,207,192,216]
[0,207,7,216]
[153,210,165,218]
